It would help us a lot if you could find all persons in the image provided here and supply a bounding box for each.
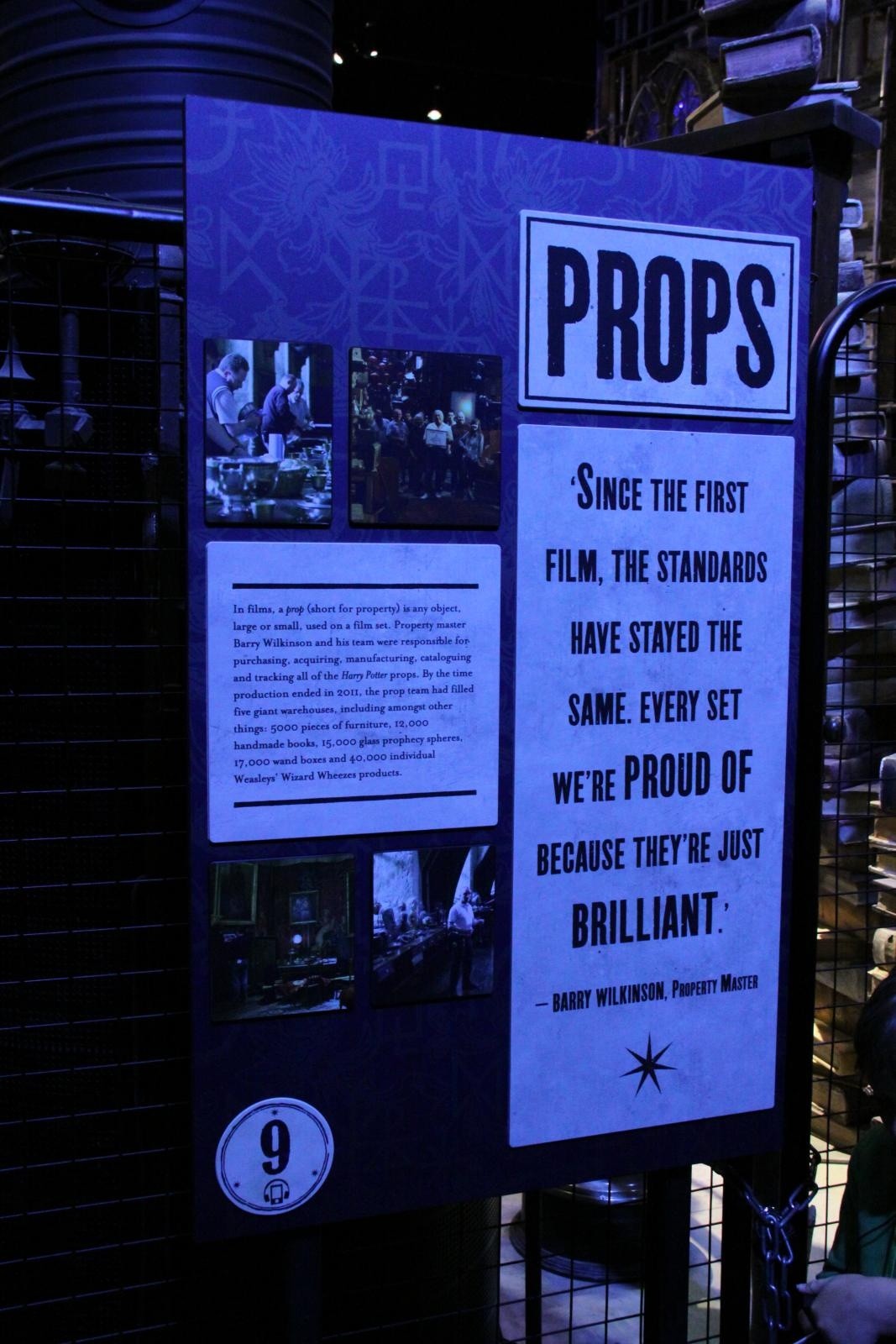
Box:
[206,350,328,471]
[352,355,497,524]
[371,888,490,994]
[801,968,896,1342]
